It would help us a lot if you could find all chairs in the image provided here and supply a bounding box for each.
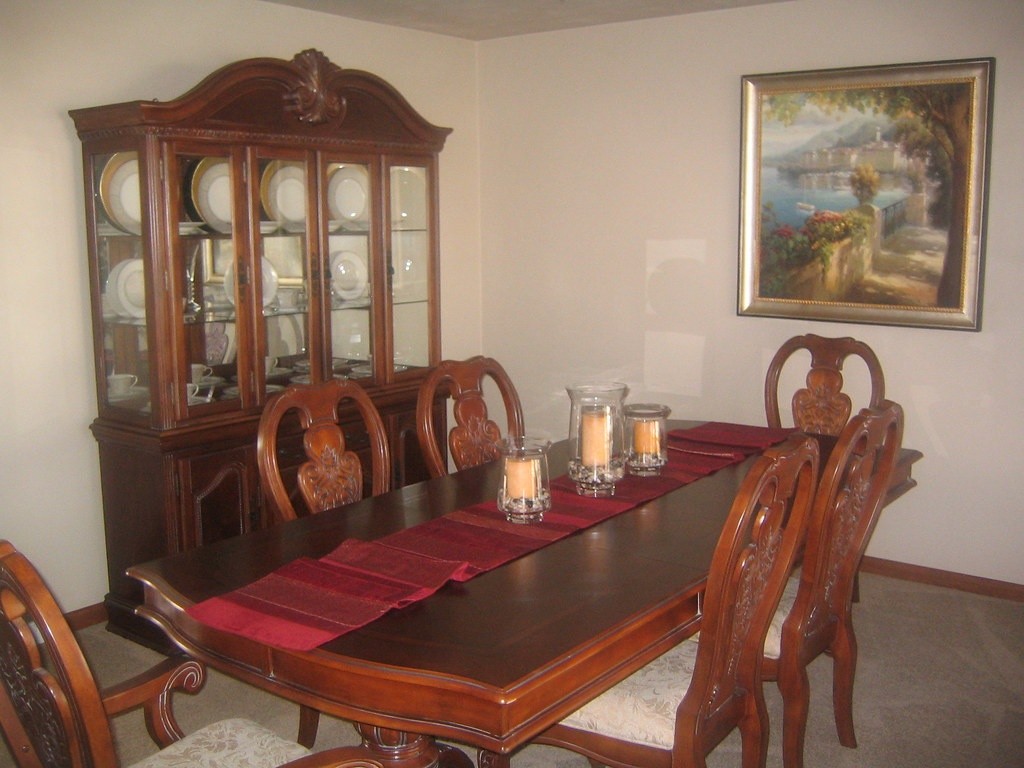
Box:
[414,353,536,483]
[700,400,907,749]
[526,428,821,768]
[762,333,888,442]
[0,539,386,768]
[257,380,407,750]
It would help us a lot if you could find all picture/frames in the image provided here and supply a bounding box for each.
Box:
[737,54,998,329]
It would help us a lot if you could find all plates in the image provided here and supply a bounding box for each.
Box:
[118,260,146,318]
[99,150,428,234]
[330,251,368,300]
[224,256,279,309]
[106,260,131,317]
[107,358,408,414]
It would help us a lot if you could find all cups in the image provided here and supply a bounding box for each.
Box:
[108,374,138,396]
[565,380,628,498]
[191,364,213,384]
[622,405,671,477]
[496,434,552,524]
[265,356,279,375]
[186,384,199,406]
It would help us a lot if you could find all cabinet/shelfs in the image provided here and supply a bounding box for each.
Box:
[65,46,453,666]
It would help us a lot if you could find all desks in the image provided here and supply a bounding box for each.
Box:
[122,400,924,767]
